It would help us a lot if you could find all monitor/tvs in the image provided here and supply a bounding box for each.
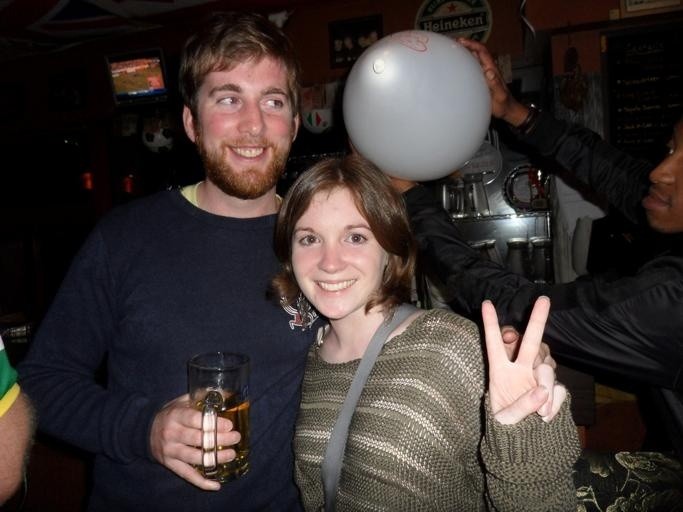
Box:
[102,45,174,106]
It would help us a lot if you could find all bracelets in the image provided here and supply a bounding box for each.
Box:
[512,100,540,135]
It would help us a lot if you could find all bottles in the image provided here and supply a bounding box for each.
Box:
[460,172,491,218]
[465,235,554,282]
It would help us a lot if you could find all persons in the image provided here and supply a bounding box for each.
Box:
[263,154,582,511]
[390,34,682,462]
[0,335,39,505]
[19,14,557,512]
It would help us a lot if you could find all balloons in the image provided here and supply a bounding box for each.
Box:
[342,29,492,180]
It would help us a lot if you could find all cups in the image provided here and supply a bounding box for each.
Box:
[187,351,251,482]
